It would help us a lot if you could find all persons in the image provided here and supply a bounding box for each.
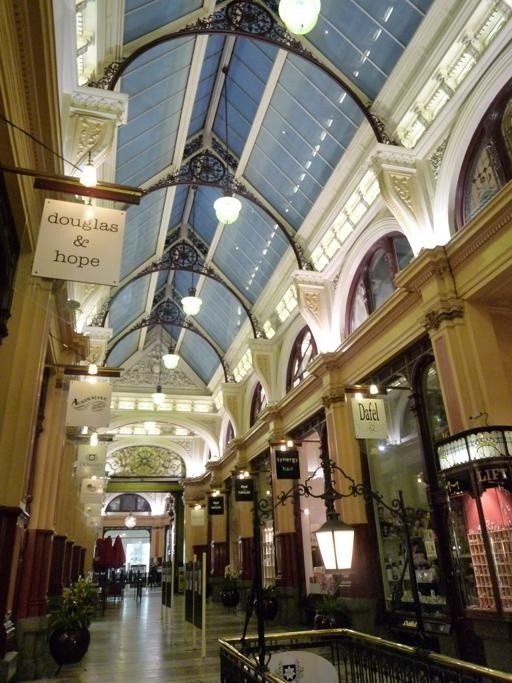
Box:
[149,563,158,587]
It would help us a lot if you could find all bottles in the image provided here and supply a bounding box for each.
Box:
[380,518,446,604]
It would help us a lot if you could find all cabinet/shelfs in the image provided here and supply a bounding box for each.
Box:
[383,532,454,637]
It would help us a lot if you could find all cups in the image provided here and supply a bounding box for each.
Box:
[309,576,316,583]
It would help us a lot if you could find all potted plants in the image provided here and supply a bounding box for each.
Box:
[221,575,240,608]
[46,594,90,665]
[254,584,280,621]
[314,577,344,630]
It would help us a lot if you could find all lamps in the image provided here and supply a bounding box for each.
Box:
[180,187,203,314]
[278,0,321,36]
[313,499,356,574]
[151,373,166,404]
[214,67,242,224]
[163,325,179,371]
[79,150,96,186]
[125,493,136,528]
